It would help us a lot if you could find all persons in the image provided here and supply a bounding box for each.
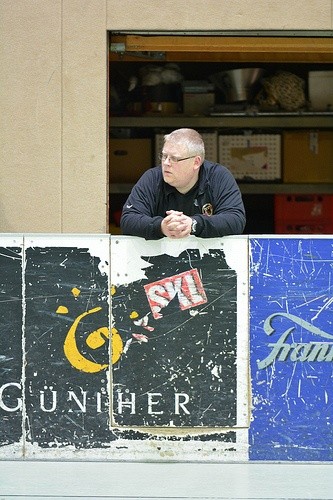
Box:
[121,129,246,239]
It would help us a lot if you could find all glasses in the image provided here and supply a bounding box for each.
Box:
[158,152,196,163]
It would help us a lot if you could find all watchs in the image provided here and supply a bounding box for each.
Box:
[190,218,197,235]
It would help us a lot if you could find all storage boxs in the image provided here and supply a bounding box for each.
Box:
[109,138,152,184]
[283,131,333,183]
[274,193,333,234]
[219,134,281,181]
[155,133,217,167]
[182,79,215,114]
